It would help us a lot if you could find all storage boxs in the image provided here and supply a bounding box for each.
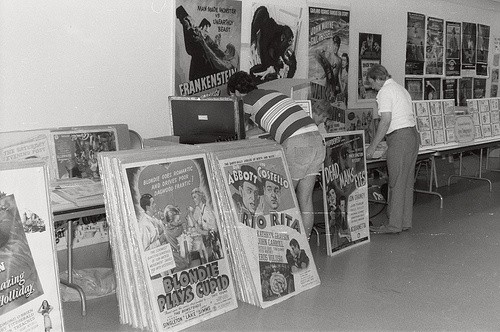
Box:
[168,95,246,145]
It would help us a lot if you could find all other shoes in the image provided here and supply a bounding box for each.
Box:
[370,225,408,235]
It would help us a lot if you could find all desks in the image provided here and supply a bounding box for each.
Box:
[50,138,500,315]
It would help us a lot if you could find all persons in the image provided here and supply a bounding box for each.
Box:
[227,71,325,241]
[322,36,348,102]
[229,136,363,273]
[366,65,419,234]
